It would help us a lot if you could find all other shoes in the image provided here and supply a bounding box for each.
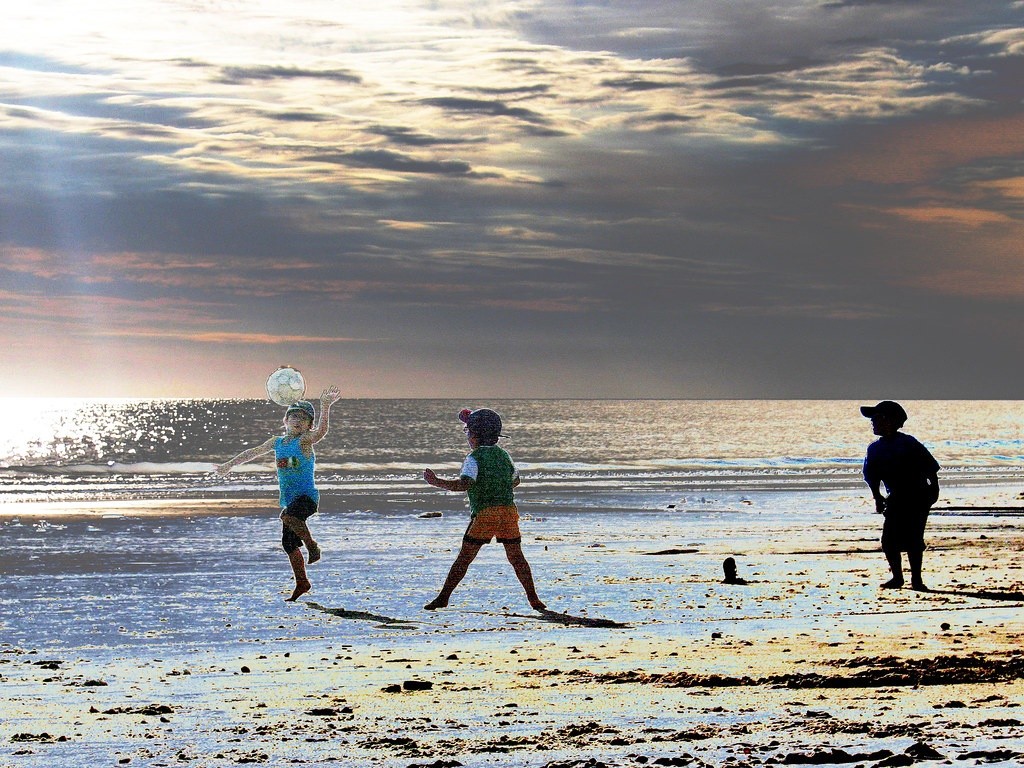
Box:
[880,577,905,588]
[911,578,922,589]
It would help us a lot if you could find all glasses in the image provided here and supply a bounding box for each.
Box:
[288,413,311,422]
[463,427,475,435]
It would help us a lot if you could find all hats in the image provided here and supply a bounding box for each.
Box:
[285,400,315,420]
[459,408,502,435]
[860,402,908,429]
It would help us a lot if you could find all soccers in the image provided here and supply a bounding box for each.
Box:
[265,367,307,407]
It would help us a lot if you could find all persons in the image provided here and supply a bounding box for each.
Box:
[860,401,941,591]
[424,408,547,610]
[211,384,341,602]
